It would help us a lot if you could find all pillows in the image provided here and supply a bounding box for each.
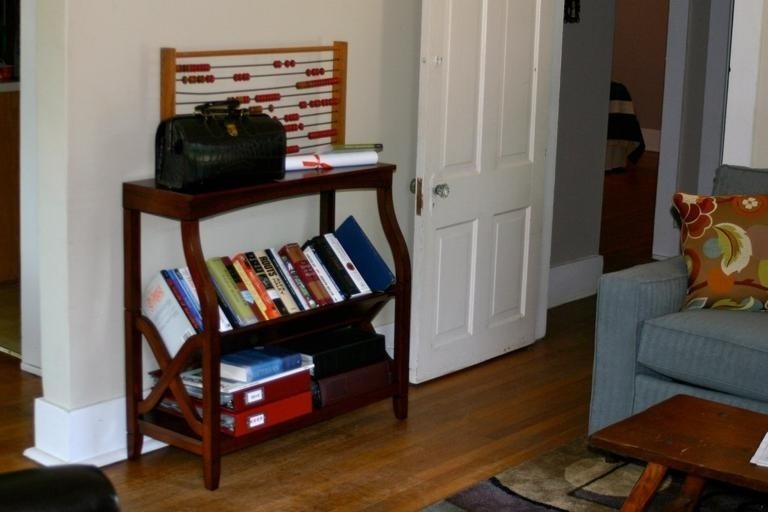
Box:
[671,193,768,312]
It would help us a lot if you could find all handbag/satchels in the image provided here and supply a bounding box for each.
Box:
[154,99,287,195]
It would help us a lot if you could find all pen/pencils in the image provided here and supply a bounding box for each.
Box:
[329,144,383,149]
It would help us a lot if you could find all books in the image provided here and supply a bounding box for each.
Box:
[139,214,398,438]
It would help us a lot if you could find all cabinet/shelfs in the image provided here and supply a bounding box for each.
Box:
[123,162,411,490]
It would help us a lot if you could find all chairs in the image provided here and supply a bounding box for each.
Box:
[587,164,767,434]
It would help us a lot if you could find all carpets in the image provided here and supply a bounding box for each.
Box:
[418,432,768,512]
